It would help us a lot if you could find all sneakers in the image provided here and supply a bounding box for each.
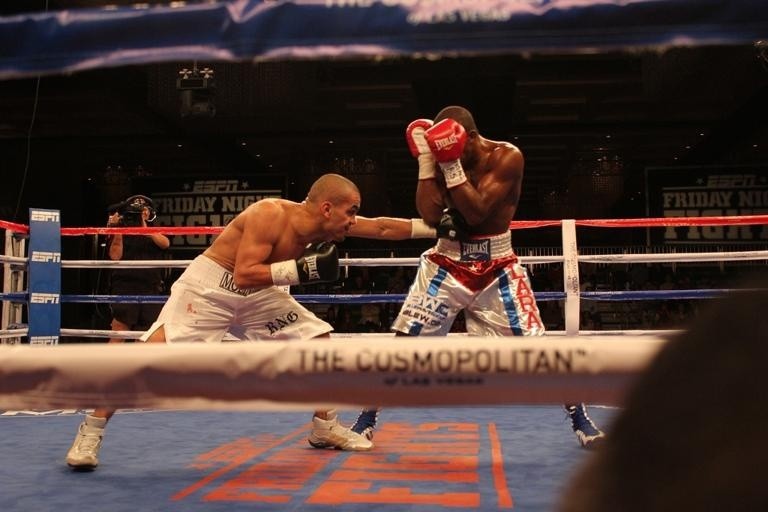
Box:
[308,410,373,452]
[566,403,604,449]
[352,409,378,439]
[67,415,108,470]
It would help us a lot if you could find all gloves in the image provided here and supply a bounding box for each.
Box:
[407,117,439,180]
[425,119,469,189]
[412,207,463,240]
[271,242,340,287]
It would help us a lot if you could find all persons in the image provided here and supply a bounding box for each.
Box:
[350,105,606,448]
[554,271,768,511]
[104,207,169,343]
[66,173,466,468]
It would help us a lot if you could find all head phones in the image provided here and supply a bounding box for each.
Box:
[122,195,160,224]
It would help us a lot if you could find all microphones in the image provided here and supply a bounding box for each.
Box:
[146,215,155,223]
